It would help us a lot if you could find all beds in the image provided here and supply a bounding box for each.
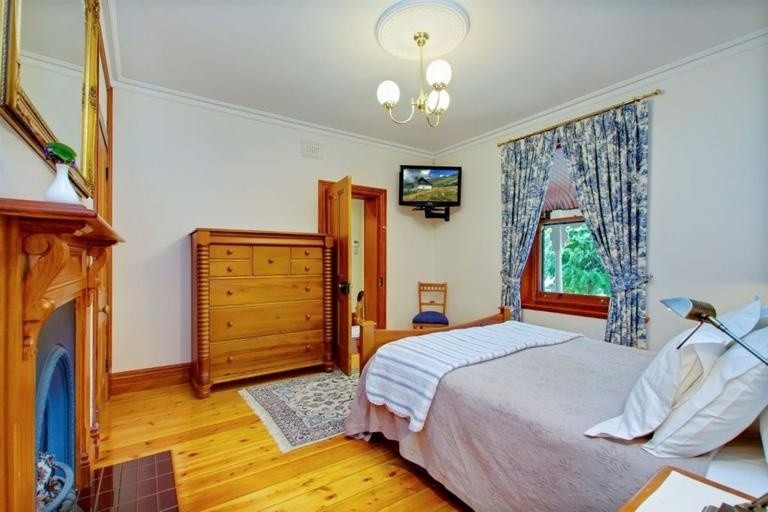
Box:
[359,306,768,512]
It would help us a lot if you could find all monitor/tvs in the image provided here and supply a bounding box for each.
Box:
[398,164,462,211]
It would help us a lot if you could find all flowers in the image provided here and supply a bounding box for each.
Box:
[44,142,76,167]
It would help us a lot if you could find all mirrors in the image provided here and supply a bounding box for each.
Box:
[2,0,100,198]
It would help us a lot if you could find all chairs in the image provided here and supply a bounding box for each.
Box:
[412,282,448,330]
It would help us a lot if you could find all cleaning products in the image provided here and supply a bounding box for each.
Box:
[41,141,78,203]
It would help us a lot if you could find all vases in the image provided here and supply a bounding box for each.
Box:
[46,163,79,203]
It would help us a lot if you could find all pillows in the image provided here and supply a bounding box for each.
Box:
[584,296,761,440]
[641,326,768,458]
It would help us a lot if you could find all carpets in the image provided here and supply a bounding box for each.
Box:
[238,368,359,453]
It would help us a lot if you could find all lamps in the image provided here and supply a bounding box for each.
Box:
[374,0,471,128]
[660,297,768,367]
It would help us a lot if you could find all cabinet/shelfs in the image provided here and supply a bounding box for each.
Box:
[189,227,335,399]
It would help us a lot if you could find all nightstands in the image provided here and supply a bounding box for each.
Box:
[618,465,768,512]
